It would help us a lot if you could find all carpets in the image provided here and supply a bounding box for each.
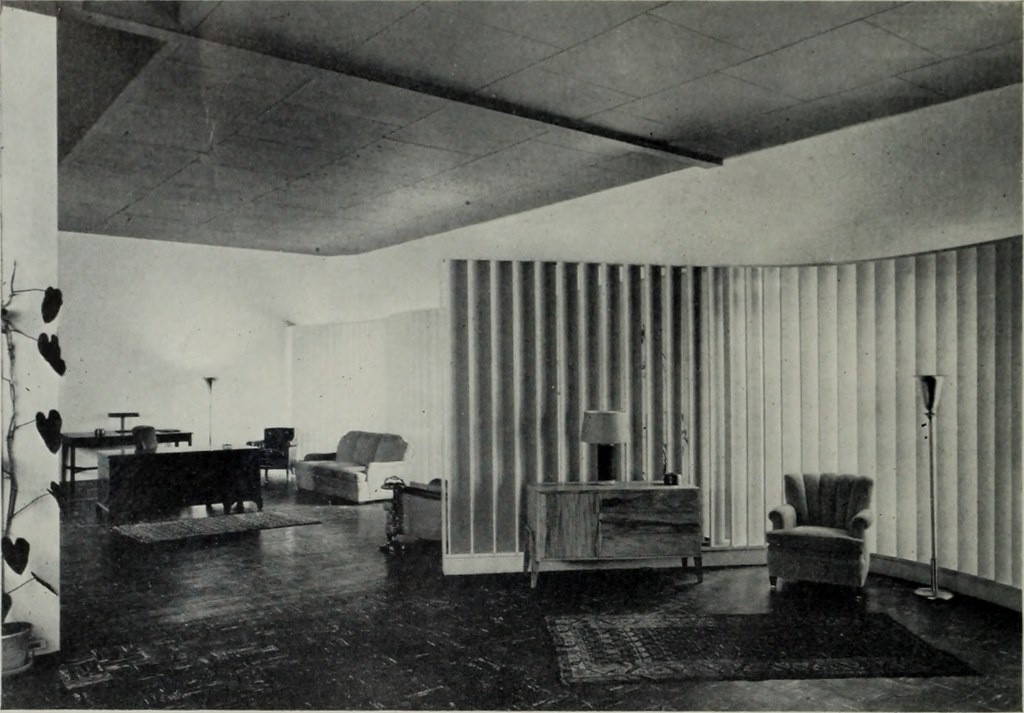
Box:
[112,508,321,544]
[542,609,976,685]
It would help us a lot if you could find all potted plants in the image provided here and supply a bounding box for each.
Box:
[0,262,66,684]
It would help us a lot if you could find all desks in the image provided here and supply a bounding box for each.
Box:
[94,449,264,521]
[58,431,193,494]
[525,481,708,586]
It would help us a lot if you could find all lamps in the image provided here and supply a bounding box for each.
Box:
[916,373,948,605]
[204,377,218,448]
[580,410,629,485]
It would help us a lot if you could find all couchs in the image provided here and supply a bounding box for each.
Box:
[765,473,876,598]
[294,429,413,504]
[384,481,447,553]
[248,427,298,478]
